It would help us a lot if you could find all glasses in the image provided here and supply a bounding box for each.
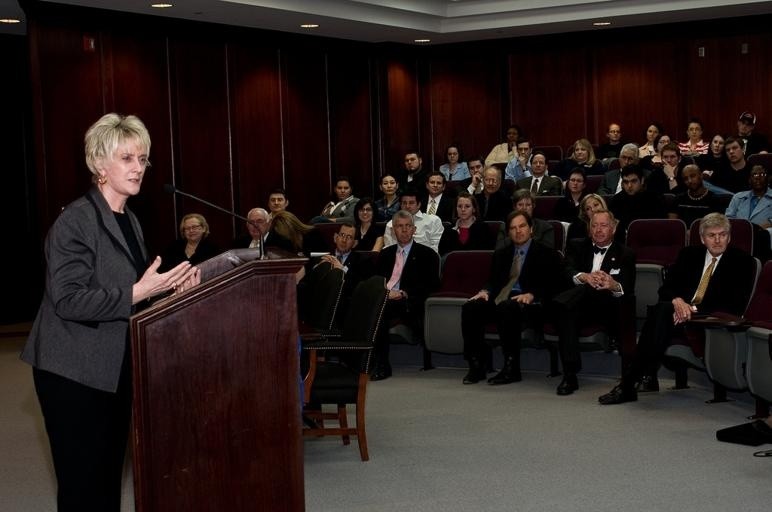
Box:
[183,225,201,230]
[751,173,767,178]
[360,208,372,212]
[338,233,354,241]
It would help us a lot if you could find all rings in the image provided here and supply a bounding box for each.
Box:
[175,281,177,288]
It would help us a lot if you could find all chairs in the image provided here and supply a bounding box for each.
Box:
[622,219,686,318]
[484,221,504,243]
[444,179,463,194]
[492,162,507,179]
[302,275,390,461]
[532,146,563,162]
[297,268,344,332]
[532,196,564,220]
[608,158,621,172]
[726,260,772,419]
[484,250,564,375]
[547,221,565,253]
[747,154,772,186]
[599,194,613,206]
[503,179,516,194]
[303,223,343,251]
[418,249,493,372]
[682,156,694,167]
[543,330,606,377]
[548,161,565,180]
[355,251,381,270]
[376,222,386,235]
[714,194,733,215]
[688,218,753,255]
[443,222,452,229]
[389,247,441,348]
[665,257,762,404]
[585,175,604,194]
[566,144,598,159]
[663,194,677,205]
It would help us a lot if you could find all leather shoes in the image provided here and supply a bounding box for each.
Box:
[489,369,522,385]
[599,384,638,404]
[464,370,487,384]
[634,376,660,392]
[556,377,579,395]
[716,422,772,446]
[371,367,393,382]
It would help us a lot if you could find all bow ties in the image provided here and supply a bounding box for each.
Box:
[593,246,606,255]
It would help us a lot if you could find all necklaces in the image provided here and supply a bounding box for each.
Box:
[360,225,366,235]
[687,188,709,200]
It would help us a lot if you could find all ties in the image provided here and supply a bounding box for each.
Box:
[338,256,344,263]
[691,258,717,304]
[494,252,522,306]
[386,248,404,289]
[531,179,539,196]
[476,179,482,194]
[252,240,259,248]
[325,205,334,216]
[428,200,436,214]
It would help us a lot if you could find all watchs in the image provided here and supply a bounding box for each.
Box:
[399,290,404,297]
[522,167,528,172]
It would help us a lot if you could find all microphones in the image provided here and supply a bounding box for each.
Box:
[163,184,264,261]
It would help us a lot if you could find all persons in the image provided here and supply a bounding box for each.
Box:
[354,197,385,253]
[484,126,527,164]
[399,150,431,206]
[375,171,402,213]
[608,140,642,171]
[458,155,491,197]
[462,210,560,386]
[654,131,674,158]
[368,210,441,381]
[266,189,289,216]
[270,212,318,272]
[497,188,556,255]
[384,190,445,254]
[667,162,717,220]
[418,168,455,225]
[472,162,513,220]
[638,121,658,155]
[510,139,538,180]
[562,170,591,210]
[726,110,771,159]
[722,133,755,185]
[438,190,497,255]
[566,192,609,240]
[557,136,604,178]
[651,144,689,179]
[19,112,202,512]
[598,120,641,162]
[706,128,735,165]
[173,212,226,267]
[237,208,270,251]
[599,211,757,405]
[557,208,639,395]
[517,152,562,194]
[438,140,472,183]
[725,162,772,227]
[605,163,666,223]
[308,177,364,227]
[308,221,366,335]
[677,113,708,160]
